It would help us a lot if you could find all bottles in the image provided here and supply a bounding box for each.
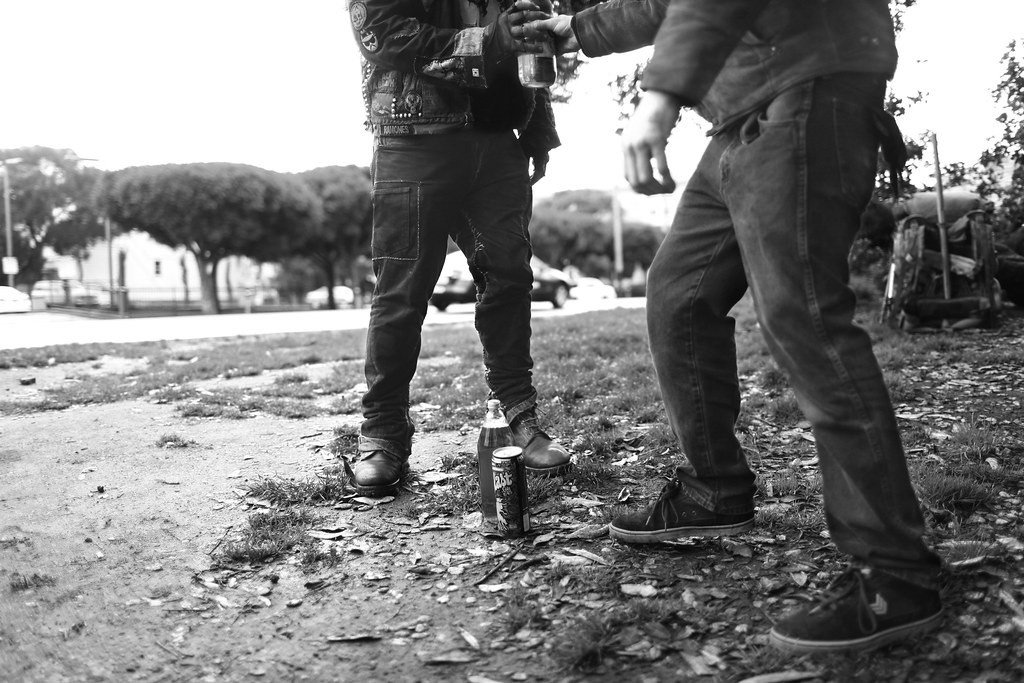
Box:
[478,399,516,523]
[512,0,555,88]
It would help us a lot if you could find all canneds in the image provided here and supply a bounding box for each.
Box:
[517,0,557,88]
[492,446,531,538]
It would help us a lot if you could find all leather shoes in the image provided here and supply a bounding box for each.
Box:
[356,450,410,498]
[510,403,572,478]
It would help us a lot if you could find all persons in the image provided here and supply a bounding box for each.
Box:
[347,0,572,496]
[511,0,954,654]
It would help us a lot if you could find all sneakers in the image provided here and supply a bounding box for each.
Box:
[769,559,944,656]
[609,477,755,543]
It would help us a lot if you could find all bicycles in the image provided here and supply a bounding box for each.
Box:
[879,249,908,330]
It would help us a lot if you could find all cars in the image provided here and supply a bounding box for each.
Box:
[570,276,618,303]
[429,248,575,311]
[303,285,356,309]
[31,279,99,310]
[3,286,31,313]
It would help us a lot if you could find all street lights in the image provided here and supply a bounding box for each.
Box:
[1,156,26,286]
